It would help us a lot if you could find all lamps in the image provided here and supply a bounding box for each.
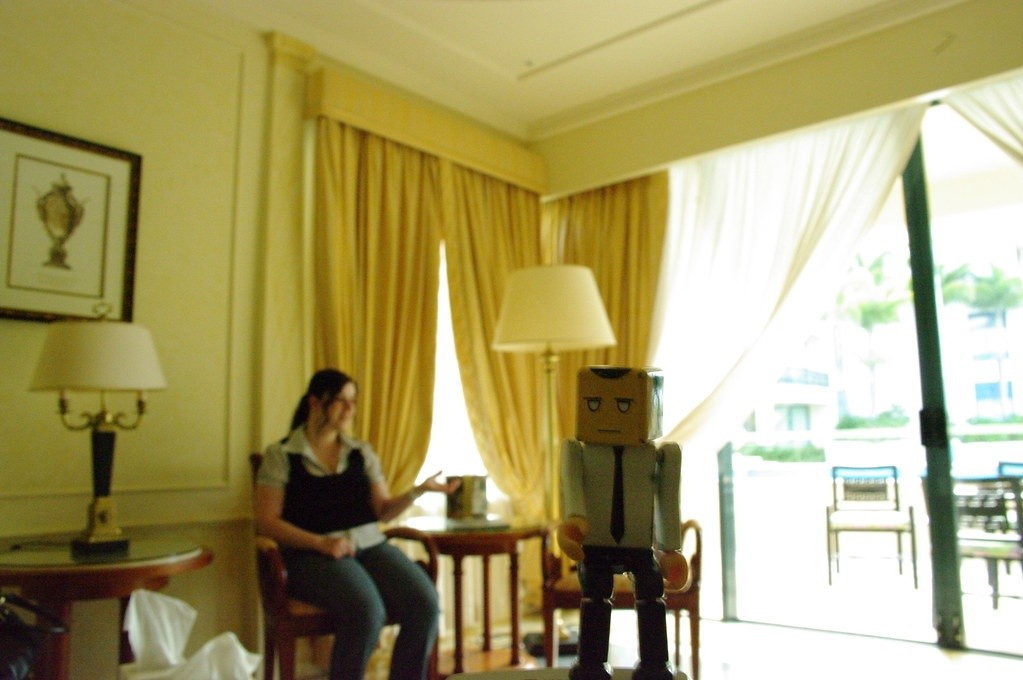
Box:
[26,323,168,555]
[492,263,619,658]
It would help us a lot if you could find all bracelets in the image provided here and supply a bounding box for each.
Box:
[407,487,422,499]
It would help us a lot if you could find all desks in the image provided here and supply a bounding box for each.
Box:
[0,533,215,680]
[398,514,550,674]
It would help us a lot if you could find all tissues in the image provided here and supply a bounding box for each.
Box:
[118,587,262,680]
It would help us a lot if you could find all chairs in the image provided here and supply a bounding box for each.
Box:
[824,466,919,591]
[244,452,439,680]
[919,462,1023,610]
[543,520,701,680]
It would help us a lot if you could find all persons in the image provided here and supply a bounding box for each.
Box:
[252,367,462,680]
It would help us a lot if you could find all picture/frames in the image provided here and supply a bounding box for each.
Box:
[0,116,141,323]
